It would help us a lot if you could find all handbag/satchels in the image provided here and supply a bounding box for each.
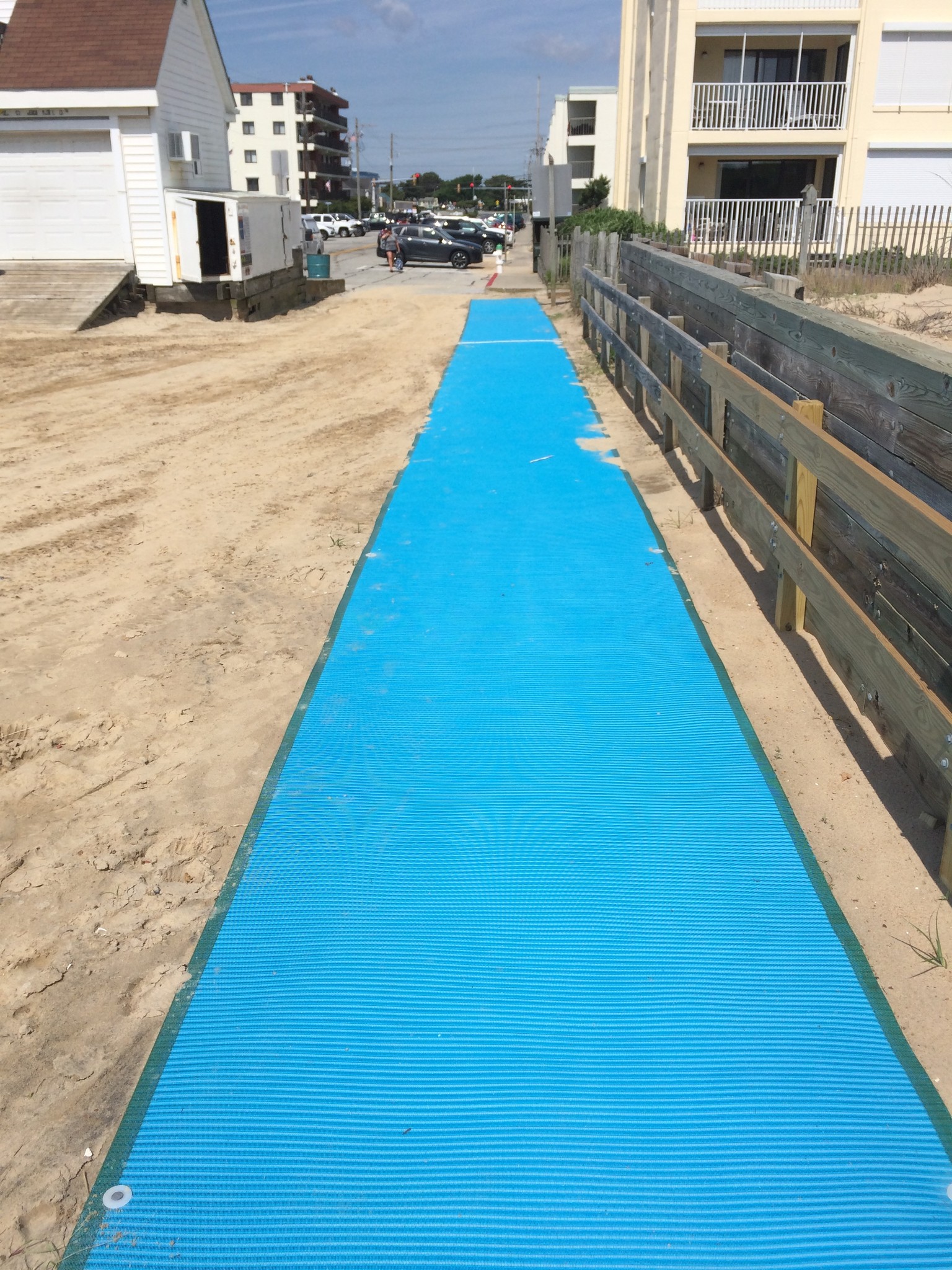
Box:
[381,237,386,249]
[394,252,403,270]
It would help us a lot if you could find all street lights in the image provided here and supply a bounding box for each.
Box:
[303,132,326,214]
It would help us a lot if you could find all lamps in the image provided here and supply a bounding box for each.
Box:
[703,51,707,59]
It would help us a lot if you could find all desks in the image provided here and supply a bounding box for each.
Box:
[704,100,737,105]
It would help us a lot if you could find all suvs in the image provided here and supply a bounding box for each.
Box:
[301,212,526,269]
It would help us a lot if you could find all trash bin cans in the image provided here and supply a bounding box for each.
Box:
[306,254,330,279]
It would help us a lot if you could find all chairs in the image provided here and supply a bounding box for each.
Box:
[728,100,757,127]
[784,90,839,130]
[693,105,714,127]
[694,204,725,241]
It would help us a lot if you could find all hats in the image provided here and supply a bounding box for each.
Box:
[387,226,391,230]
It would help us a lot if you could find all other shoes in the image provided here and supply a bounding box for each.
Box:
[390,269,393,272]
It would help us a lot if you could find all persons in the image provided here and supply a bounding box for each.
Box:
[382,226,400,273]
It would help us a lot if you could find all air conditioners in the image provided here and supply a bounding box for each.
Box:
[167,130,201,161]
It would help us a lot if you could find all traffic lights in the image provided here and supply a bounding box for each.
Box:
[413,173,420,186]
[508,184,512,189]
[470,182,474,188]
